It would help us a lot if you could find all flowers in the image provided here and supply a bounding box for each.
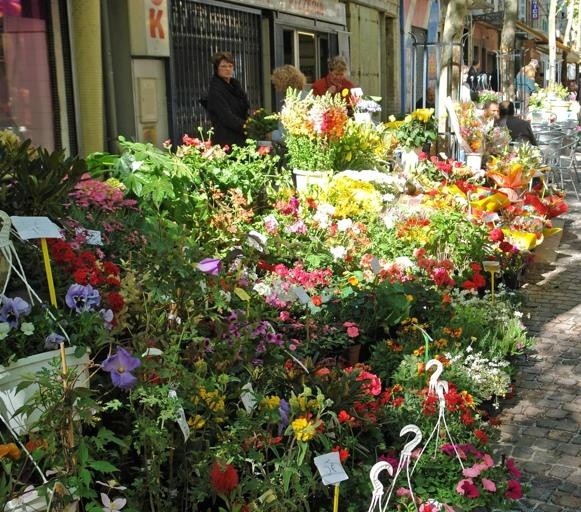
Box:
[0,81,581,512]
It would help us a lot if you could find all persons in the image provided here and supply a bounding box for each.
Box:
[307,55,359,114]
[515,59,539,105]
[206,50,251,163]
[481,99,543,191]
[462,65,472,102]
[416,86,435,127]
[467,58,480,93]
[266,66,314,144]
